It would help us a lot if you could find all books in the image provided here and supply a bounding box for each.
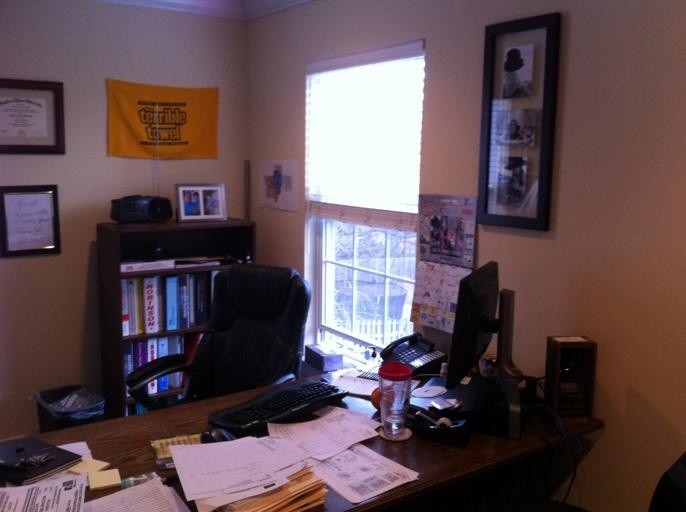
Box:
[0,435,83,488]
[121,256,224,416]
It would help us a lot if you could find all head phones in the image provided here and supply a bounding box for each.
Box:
[415,406,466,442]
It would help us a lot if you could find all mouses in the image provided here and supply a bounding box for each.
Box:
[200,427,235,444]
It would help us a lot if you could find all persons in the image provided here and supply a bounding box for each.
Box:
[504,155,528,192]
[184,190,221,216]
[503,117,524,140]
[500,47,531,99]
[421,205,467,257]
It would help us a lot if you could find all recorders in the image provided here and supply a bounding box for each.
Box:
[111,195,172,226]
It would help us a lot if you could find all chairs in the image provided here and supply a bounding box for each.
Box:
[505,449,686,511]
[127,259,312,412]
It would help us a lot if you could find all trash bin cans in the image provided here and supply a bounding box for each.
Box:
[35,384,106,434]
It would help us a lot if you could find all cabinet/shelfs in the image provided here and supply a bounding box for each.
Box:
[92,215,259,423]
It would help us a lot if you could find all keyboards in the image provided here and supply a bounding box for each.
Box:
[208,380,349,434]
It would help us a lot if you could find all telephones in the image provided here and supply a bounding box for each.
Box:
[380,332,448,375]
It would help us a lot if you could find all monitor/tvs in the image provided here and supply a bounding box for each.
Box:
[445,261,521,439]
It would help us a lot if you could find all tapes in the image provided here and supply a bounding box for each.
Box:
[436,417,451,427]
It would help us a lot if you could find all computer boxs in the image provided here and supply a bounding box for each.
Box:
[409,376,508,440]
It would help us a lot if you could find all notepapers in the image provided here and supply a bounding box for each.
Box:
[89,469,122,490]
[68,459,111,475]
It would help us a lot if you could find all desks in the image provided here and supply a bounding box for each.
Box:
[0,369,608,510]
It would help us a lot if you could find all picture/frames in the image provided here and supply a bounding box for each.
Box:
[171,180,228,224]
[472,11,563,234]
[0,76,66,158]
[0,184,62,257]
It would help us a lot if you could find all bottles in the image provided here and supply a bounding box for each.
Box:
[439,362,448,377]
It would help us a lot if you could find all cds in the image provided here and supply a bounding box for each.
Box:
[412,385,447,398]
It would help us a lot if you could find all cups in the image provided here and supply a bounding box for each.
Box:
[378,362,413,437]
